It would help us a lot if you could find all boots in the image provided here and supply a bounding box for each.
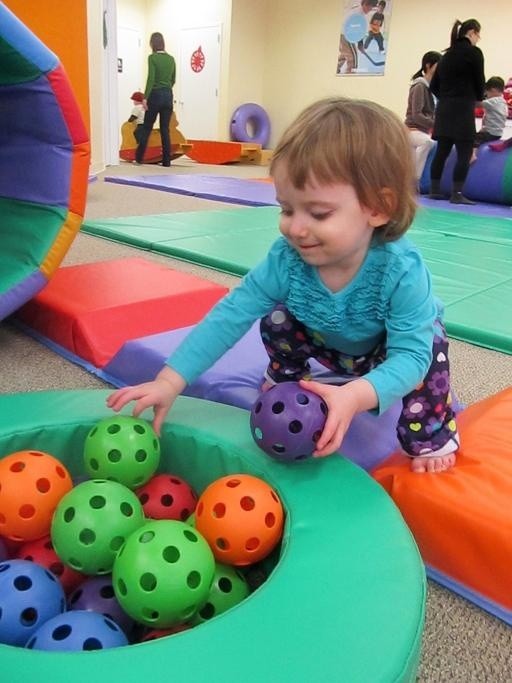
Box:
[430,178,447,199]
[450,178,478,205]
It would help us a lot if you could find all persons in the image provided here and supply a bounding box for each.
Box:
[127,92,146,144]
[107,94,461,475]
[429,18,485,205]
[405,51,443,136]
[336,0,385,75]
[469,76,509,164]
[133,32,176,166]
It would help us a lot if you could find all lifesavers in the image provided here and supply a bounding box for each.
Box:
[231,103,271,150]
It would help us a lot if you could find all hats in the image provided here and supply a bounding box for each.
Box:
[129,91,145,102]
